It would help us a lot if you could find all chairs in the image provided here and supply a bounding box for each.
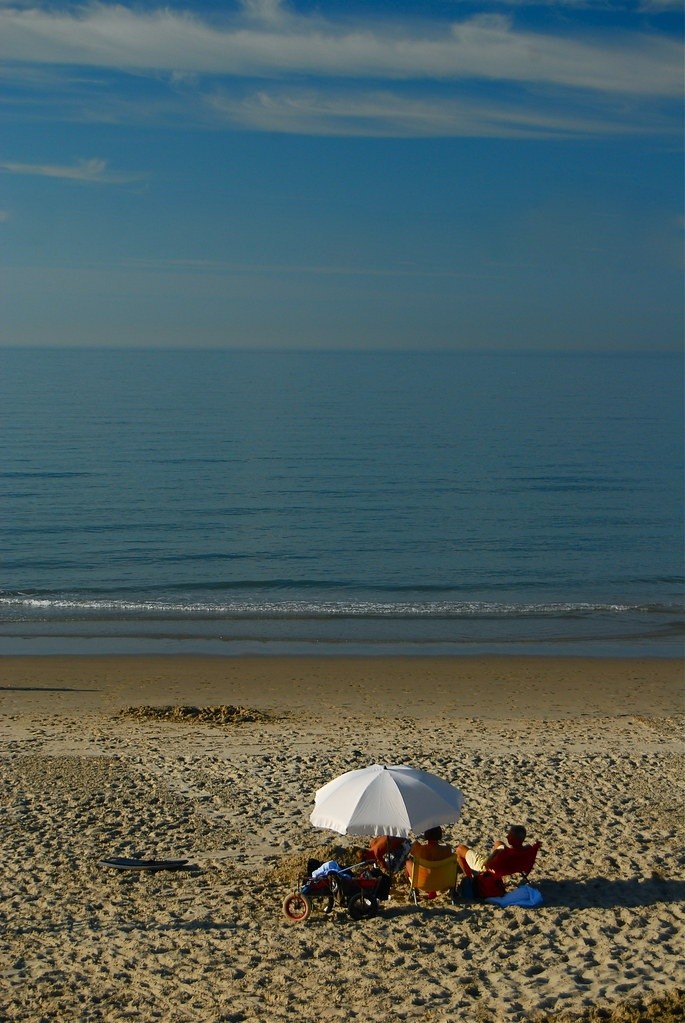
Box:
[403,842,544,910]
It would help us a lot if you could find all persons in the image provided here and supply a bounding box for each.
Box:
[457,825,527,878]
[406,825,452,898]
[356,834,412,875]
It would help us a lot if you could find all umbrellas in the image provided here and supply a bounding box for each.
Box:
[310,764,464,878]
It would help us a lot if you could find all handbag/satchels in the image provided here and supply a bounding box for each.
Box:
[458,871,506,899]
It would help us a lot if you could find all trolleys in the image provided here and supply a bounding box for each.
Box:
[282,860,394,921]
